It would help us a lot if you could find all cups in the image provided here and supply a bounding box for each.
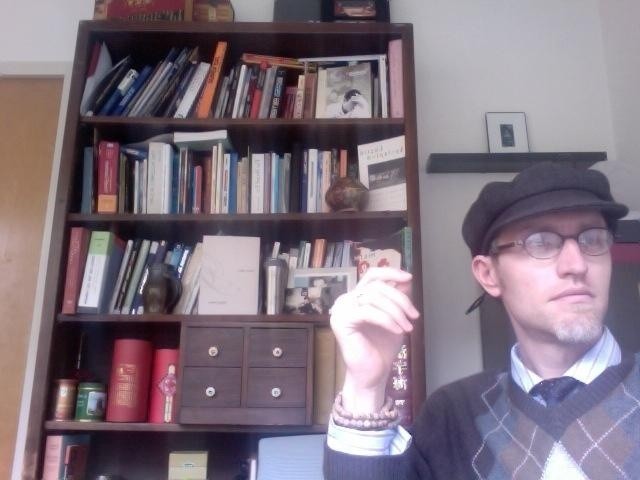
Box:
[262,258,290,316]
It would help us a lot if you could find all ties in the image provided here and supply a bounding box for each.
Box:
[530,378,581,410]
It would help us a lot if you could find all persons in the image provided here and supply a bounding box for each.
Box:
[318,152,640,479]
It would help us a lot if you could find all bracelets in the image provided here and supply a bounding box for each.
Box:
[330,392,400,430]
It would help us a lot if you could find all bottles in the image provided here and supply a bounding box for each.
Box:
[142,263,183,315]
[324,175,370,213]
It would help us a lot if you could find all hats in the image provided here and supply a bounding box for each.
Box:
[462,162,630,258]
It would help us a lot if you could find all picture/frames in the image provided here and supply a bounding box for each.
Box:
[485,110,530,154]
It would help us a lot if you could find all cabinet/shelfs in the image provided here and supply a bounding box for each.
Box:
[11,20,427,480]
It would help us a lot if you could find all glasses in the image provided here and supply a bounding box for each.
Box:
[489,228,622,259]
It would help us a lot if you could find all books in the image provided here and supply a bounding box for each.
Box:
[61,227,411,318]
[82,130,406,213]
[79,41,404,117]
